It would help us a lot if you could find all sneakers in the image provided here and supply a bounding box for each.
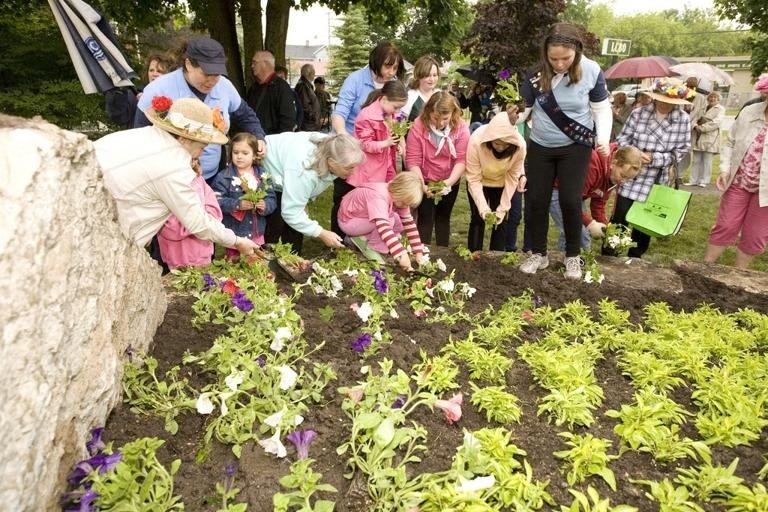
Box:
[519,253,549,274]
[350,236,385,266]
[683,183,707,187]
[563,255,581,281]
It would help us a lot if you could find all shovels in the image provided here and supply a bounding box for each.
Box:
[396,257,431,278]
[244,236,296,283]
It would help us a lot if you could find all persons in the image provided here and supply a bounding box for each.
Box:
[95,22,768,279]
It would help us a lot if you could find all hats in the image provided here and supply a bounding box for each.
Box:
[186,38,228,76]
[314,77,328,84]
[145,98,229,145]
[642,78,693,104]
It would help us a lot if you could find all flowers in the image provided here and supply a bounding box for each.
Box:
[605,223,639,258]
[231,170,272,213]
[496,70,523,103]
[653,76,689,98]
[382,113,412,139]
[152,95,173,119]
[212,107,227,131]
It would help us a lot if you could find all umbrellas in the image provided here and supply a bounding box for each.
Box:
[46,0,141,97]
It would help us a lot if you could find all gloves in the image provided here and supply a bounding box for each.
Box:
[586,219,606,240]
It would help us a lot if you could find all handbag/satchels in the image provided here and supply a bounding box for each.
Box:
[625,184,692,237]
[698,116,712,124]
